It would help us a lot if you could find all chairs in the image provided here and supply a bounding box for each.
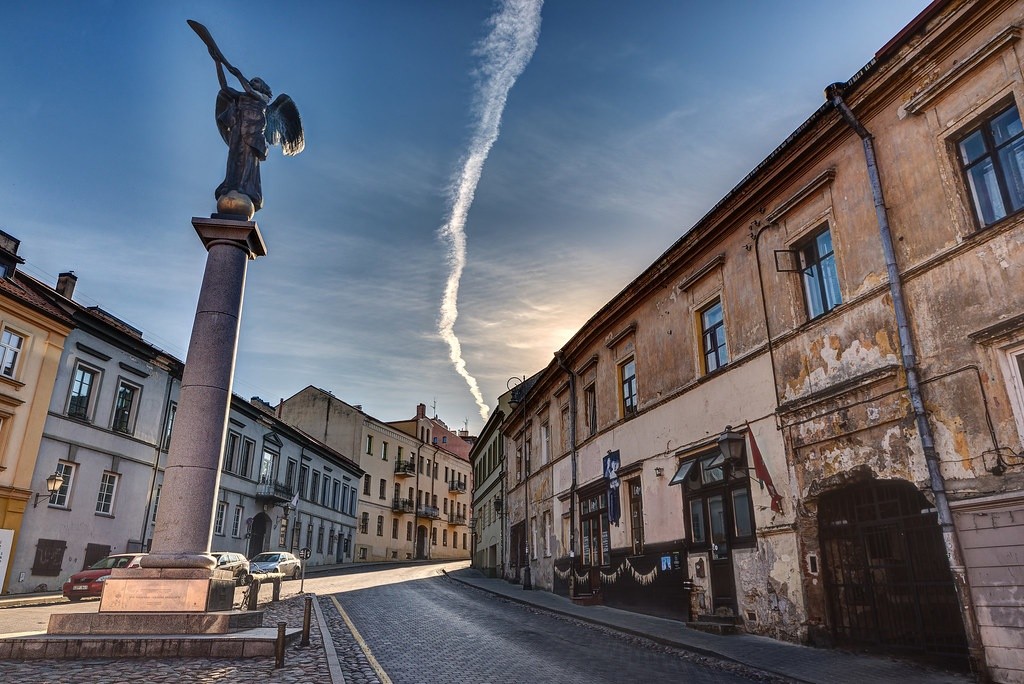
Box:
[119,561,127,568]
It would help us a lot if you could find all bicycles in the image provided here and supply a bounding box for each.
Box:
[240,563,263,610]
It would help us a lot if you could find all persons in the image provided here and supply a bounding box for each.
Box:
[214,66,305,208]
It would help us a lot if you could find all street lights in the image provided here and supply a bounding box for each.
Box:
[507,375,532,591]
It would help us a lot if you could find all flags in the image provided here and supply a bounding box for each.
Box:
[748,427,784,513]
[288,491,299,512]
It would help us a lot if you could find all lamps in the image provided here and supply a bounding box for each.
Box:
[493,495,510,516]
[715,424,766,490]
[33,471,66,509]
[276,502,291,521]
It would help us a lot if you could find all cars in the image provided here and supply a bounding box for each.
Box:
[247,552,301,580]
[63,553,149,602]
[211,552,250,586]
